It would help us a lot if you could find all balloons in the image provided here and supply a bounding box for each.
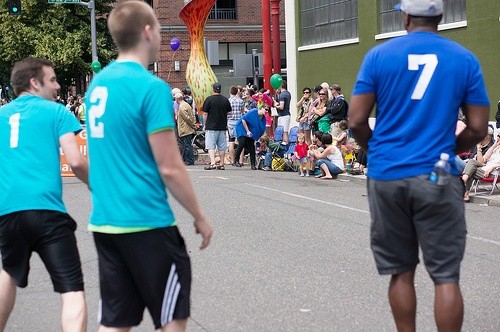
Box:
[171,38,181,51]
[270,74,282,90]
[90,61,101,73]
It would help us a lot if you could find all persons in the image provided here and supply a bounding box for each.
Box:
[0,56,88,332]
[226,79,367,179]
[455,99,500,202]
[202,82,232,170]
[85,0,214,332]
[56,95,86,124]
[348,0,489,332]
[171,86,199,165]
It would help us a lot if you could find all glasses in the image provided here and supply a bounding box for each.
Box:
[303,92,310,94]
[264,107,268,111]
[319,93,325,95]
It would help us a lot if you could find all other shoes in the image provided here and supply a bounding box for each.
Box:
[251,164,257,170]
[262,166,272,170]
[315,173,332,179]
[463,193,469,200]
[234,160,241,167]
[204,164,216,170]
[217,165,225,170]
[300,173,310,177]
[350,166,364,175]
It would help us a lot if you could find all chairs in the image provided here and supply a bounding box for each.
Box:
[260,124,300,171]
[464,131,499,195]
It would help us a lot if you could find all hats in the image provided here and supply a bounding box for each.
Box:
[488,126,493,135]
[392,0,445,16]
[330,84,341,91]
[173,93,182,99]
[213,82,221,90]
